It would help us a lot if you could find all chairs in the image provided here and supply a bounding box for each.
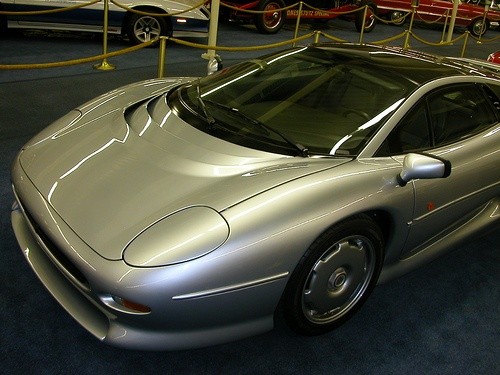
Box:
[316,65,407,122]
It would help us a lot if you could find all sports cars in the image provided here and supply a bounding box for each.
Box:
[0,1,210,48]
[8,41,500,350]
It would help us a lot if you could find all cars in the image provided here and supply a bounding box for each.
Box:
[375,0,500,39]
[219,1,379,35]
